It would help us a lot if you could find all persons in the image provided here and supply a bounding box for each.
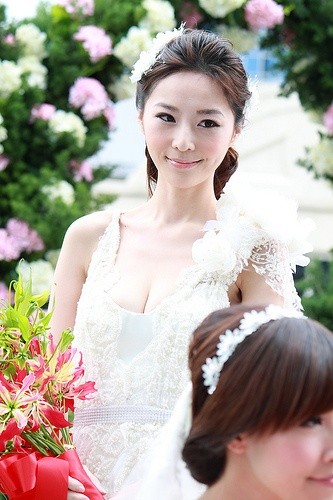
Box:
[180,304,333,500]
[45,27,305,500]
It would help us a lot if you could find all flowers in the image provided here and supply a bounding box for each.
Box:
[0,2,332,302]
[1,256,108,499]
